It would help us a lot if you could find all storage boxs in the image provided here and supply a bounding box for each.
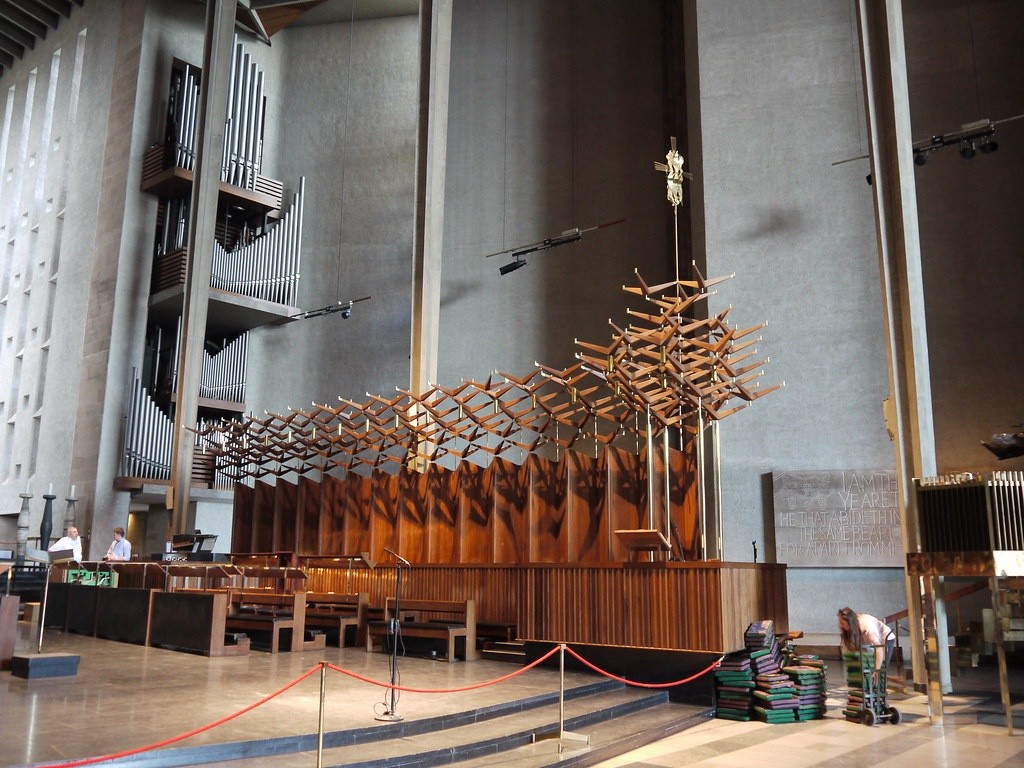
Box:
[712,620,885,725]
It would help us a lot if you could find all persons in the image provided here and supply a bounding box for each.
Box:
[104,527,131,561]
[837,606,896,687]
[48,526,83,563]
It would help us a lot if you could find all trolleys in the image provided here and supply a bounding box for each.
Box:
[846,628,903,727]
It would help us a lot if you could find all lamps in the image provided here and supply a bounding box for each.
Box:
[341,307,353,319]
[499,255,527,276]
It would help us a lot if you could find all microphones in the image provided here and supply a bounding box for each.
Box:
[383,548,410,566]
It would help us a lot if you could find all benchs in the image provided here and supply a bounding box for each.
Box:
[429,618,517,642]
[55,582,485,666]
